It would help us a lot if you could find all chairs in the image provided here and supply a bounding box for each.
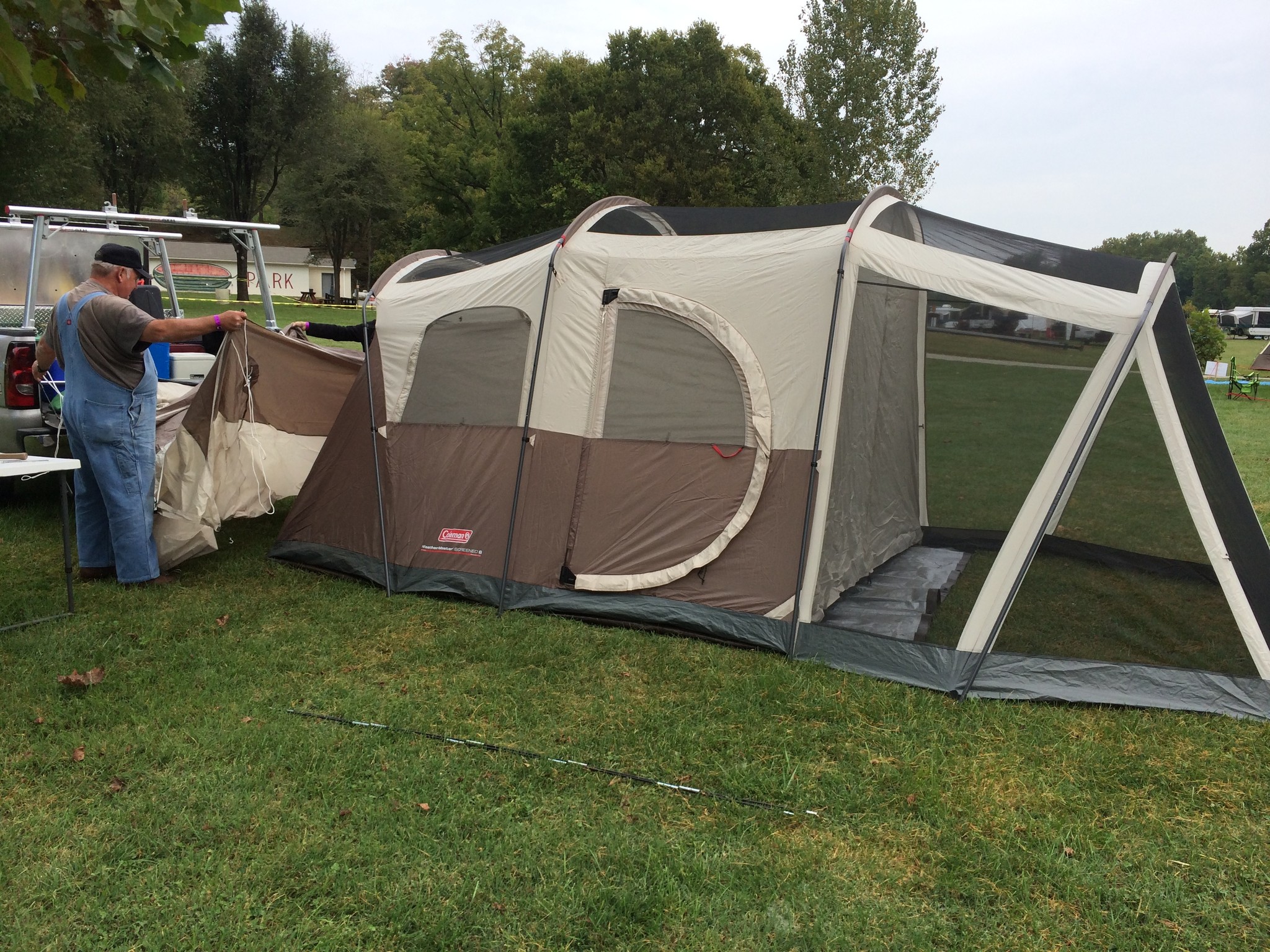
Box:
[1228,356,1260,401]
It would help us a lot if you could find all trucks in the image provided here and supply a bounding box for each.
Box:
[1201,306,1270,341]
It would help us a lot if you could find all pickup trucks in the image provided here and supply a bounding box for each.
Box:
[0,202,286,455]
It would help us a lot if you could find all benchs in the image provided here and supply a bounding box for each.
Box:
[294,298,323,304]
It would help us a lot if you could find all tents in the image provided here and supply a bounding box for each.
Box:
[269,191,1270,720]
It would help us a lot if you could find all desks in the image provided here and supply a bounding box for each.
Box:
[297,292,320,307]
[1,454,82,631]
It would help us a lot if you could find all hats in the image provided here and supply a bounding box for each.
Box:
[94,242,153,280]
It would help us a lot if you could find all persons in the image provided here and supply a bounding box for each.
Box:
[30,242,246,589]
[291,319,377,352]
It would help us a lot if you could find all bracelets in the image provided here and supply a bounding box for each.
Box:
[36,363,49,373]
[214,314,222,332]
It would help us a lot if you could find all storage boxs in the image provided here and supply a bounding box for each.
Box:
[168,351,216,380]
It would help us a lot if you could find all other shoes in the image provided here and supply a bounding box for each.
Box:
[79,565,117,582]
[126,574,176,588]
